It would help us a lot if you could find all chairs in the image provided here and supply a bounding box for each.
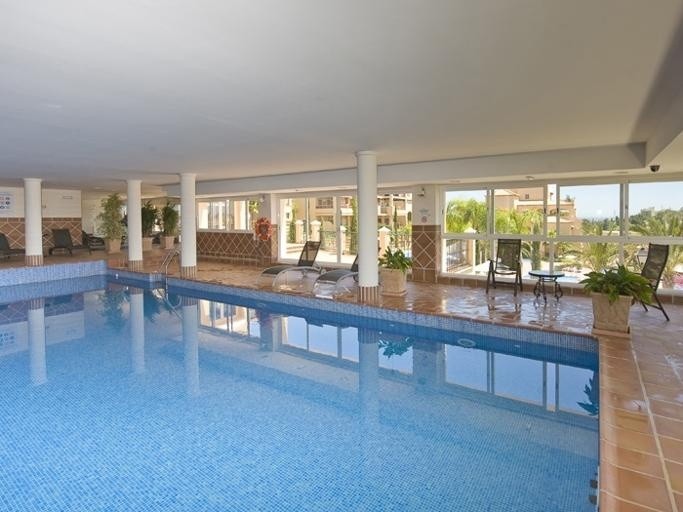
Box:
[0,232,25,259]
[256,240,321,285]
[313,247,381,300]
[632,241,670,321]
[486,238,523,296]
[48,229,74,256]
[80,229,105,254]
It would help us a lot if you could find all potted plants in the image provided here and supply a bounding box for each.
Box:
[123,198,160,251]
[96,192,126,254]
[577,260,656,338]
[158,198,179,250]
[378,245,413,297]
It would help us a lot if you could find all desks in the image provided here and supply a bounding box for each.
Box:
[529,270,565,302]
